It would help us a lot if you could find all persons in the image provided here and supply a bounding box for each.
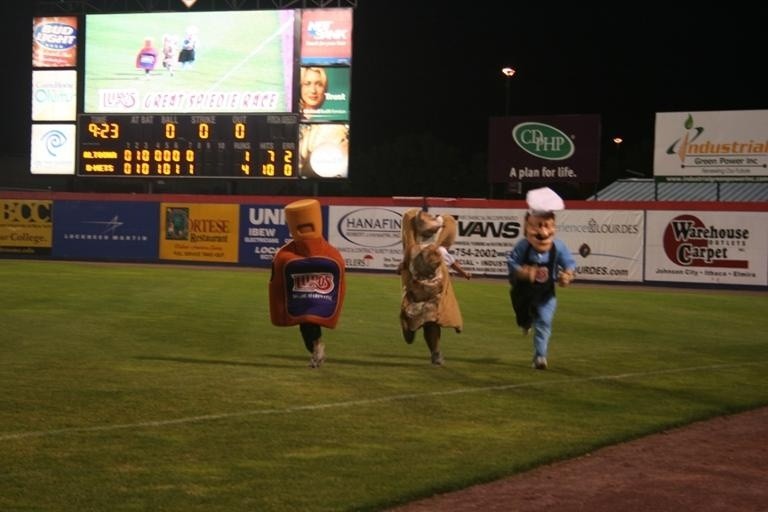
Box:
[504,186,578,371]
[172,209,188,236]
[300,67,328,111]
[394,210,471,365]
[160,37,175,77]
[268,199,346,368]
[135,40,157,73]
[179,39,196,67]
[167,208,174,238]
